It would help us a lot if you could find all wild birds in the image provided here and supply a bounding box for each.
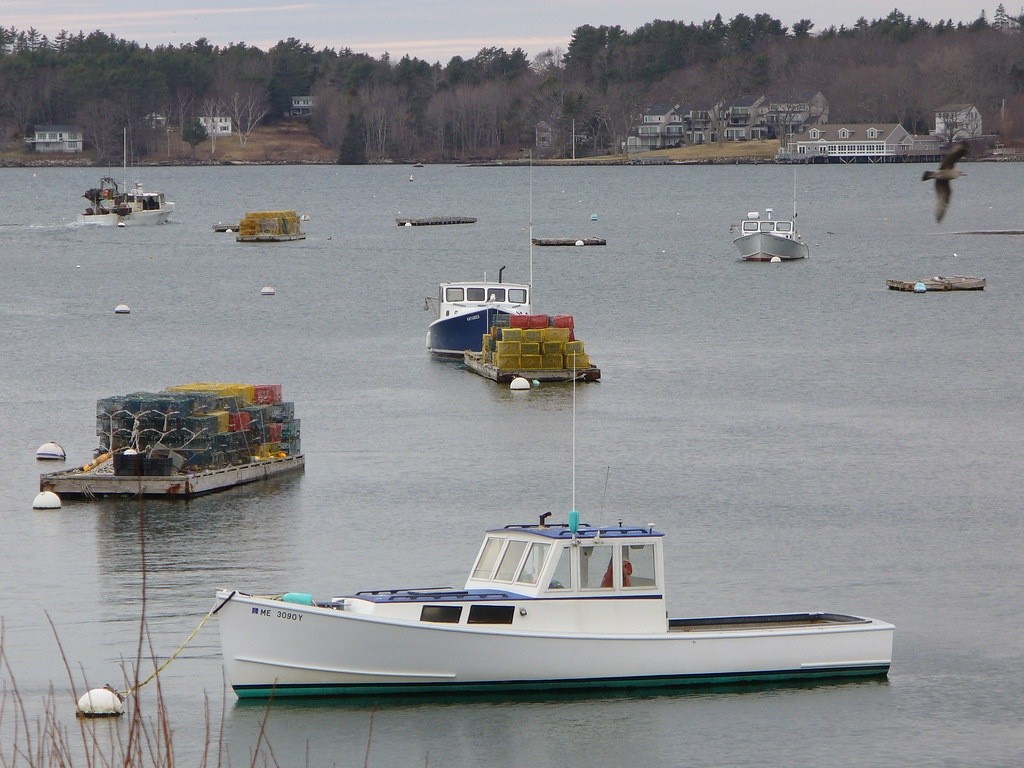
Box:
[920,143,969,223]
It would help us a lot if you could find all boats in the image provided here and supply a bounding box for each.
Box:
[732,166,807,262]
[422,266,532,362]
[78,125,174,225]
[217,348,895,700]
